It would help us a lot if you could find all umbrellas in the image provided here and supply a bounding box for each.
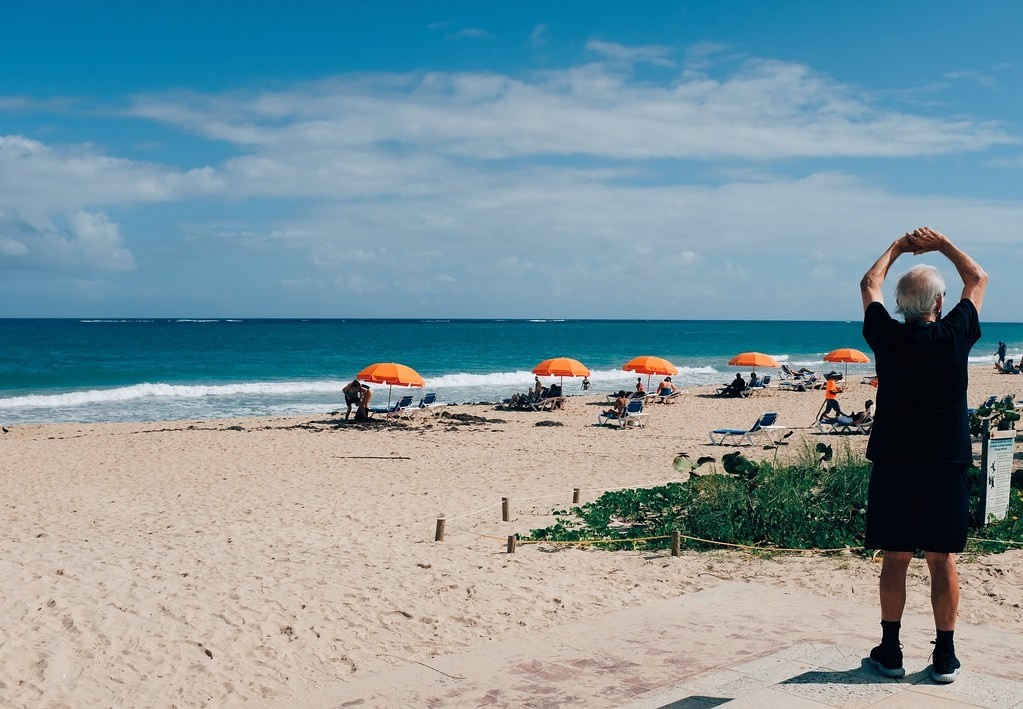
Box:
[532,357,590,403]
[823,349,870,387]
[623,355,678,389]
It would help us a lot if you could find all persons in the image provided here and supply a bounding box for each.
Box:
[356,383,373,406]
[819,371,843,420]
[749,372,756,388]
[509,374,560,411]
[1004,356,1023,374]
[859,227,988,683]
[994,342,1006,369]
[357,361,426,421]
[636,378,641,389]
[821,398,873,425]
[602,390,627,416]
[342,379,366,420]
[786,365,814,375]
[656,378,676,402]
[720,373,745,395]
[728,352,779,387]
[581,376,590,390]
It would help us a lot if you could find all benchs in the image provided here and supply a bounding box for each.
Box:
[995,362,1023,375]
[407,391,447,420]
[365,395,413,423]
[497,388,554,413]
[715,364,879,436]
[966,395,999,426]
[1012,399,1023,418]
[521,386,566,411]
[597,398,651,429]
[644,390,689,406]
[605,391,659,406]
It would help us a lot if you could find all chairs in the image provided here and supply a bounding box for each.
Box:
[707,411,787,448]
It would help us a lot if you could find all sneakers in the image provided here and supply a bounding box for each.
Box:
[869,643,905,677]
[930,646,962,682]
